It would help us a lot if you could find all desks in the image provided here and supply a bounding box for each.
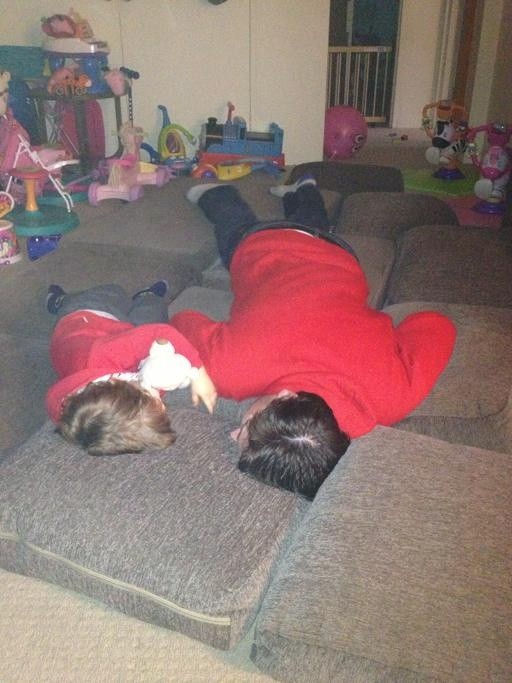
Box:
[26,87,128,176]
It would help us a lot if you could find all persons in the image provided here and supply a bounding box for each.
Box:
[170,174,458,498]
[44,281,219,457]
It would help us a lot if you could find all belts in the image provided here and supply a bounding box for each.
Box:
[254,224,345,248]
